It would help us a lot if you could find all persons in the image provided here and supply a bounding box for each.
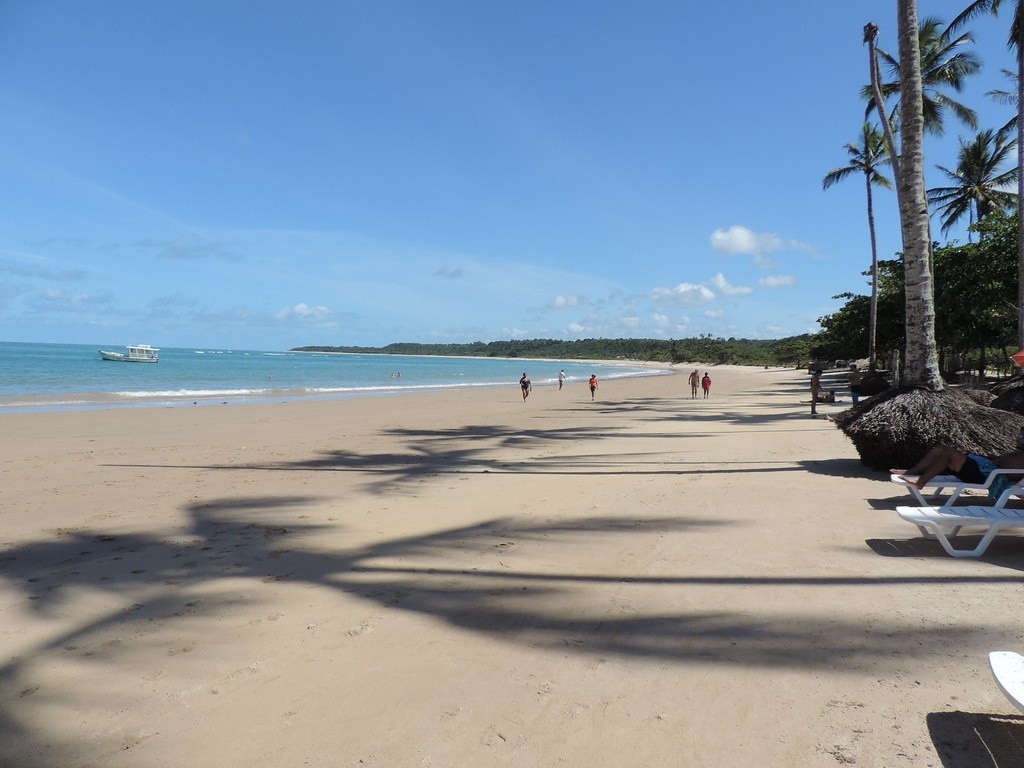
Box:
[558,369,566,390]
[810,369,823,414]
[800,390,835,403]
[688,369,700,399]
[849,364,861,408]
[890,427,1024,490]
[702,372,712,399]
[519,372,532,402]
[589,373,598,401]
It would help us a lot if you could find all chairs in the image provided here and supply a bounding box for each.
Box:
[891,468,1024,557]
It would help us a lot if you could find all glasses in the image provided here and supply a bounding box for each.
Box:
[1018,434,1024,440]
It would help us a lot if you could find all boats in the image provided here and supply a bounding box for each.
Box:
[98,343,161,363]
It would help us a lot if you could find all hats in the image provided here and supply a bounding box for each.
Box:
[592,373,595,377]
